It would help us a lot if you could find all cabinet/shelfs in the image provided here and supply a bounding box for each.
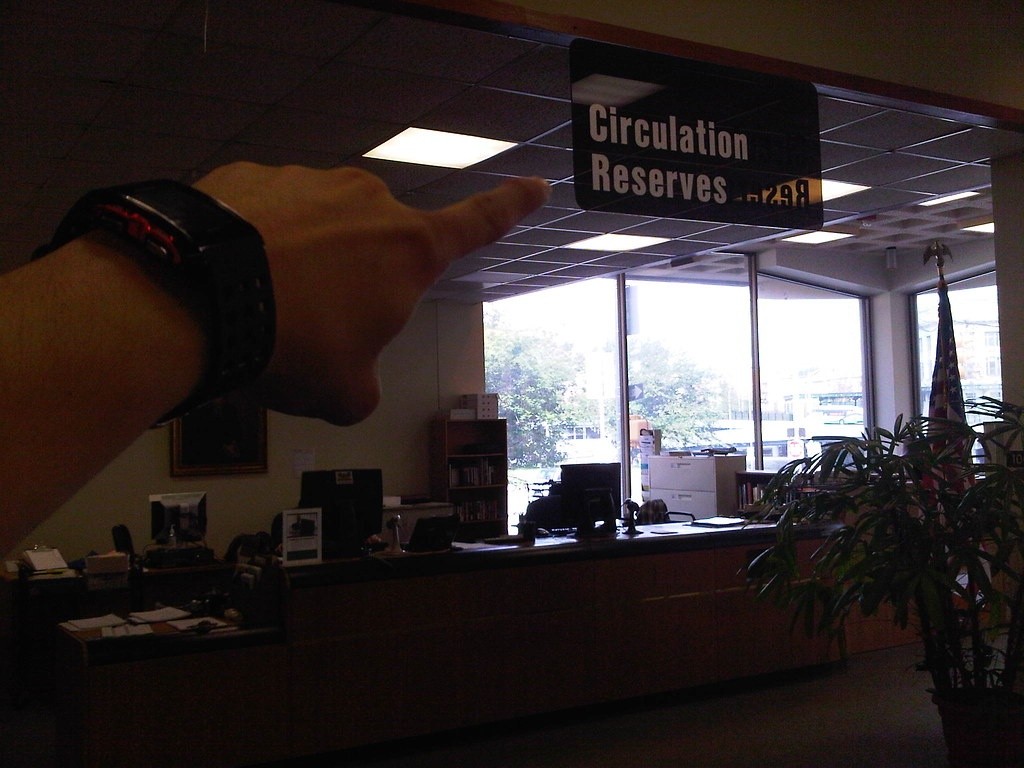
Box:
[426,418,508,537]
[647,453,747,520]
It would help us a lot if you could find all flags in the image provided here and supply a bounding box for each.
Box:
[922,279,992,609]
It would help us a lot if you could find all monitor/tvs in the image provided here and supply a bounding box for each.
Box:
[300,469,383,560]
[559,464,621,540]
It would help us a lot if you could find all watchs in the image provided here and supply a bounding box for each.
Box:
[29,178,275,428]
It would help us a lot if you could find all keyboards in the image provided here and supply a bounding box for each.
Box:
[551,527,577,535]
[359,543,390,553]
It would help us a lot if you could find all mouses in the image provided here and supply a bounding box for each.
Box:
[536,528,549,538]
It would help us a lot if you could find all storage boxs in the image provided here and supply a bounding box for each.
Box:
[86,551,129,574]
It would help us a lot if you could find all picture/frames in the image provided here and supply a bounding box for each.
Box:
[283,506,322,568]
[169,405,272,476]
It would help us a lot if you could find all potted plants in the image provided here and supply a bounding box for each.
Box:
[736,395,1024,768]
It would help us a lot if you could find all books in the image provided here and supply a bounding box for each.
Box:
[448,462,498,522]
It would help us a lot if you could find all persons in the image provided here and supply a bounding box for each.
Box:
[0,161,551,580]
